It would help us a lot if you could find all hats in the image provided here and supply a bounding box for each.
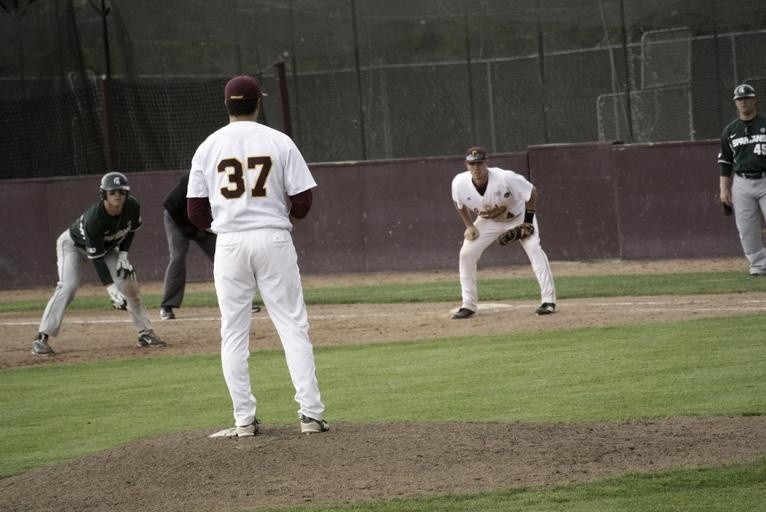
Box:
[732,84,756,100]
[225,75,268,103]
[464,147,487,165]
[100,172,130,191]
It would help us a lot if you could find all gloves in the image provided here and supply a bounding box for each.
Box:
[108,285,127,311]
[117,252,135,279]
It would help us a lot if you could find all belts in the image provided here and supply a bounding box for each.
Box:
[735,172,766,179]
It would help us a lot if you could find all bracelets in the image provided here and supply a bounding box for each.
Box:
[524,208,534,223]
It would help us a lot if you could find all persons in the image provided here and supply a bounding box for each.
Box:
[716,83,766,276]
[449,146,556,319]
[186,75,329,438]
[28,172,167,358]
[159,171,260,320]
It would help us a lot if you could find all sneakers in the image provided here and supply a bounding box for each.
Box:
[32,339,55,357]
[236,417,261,437]
[749,267,766,275]
[160,306,175,320]
[301,416,329,434]
[252,307,261,313]
[535,303,556,314]
[453,308,475,319]
[137,330,166,348]
